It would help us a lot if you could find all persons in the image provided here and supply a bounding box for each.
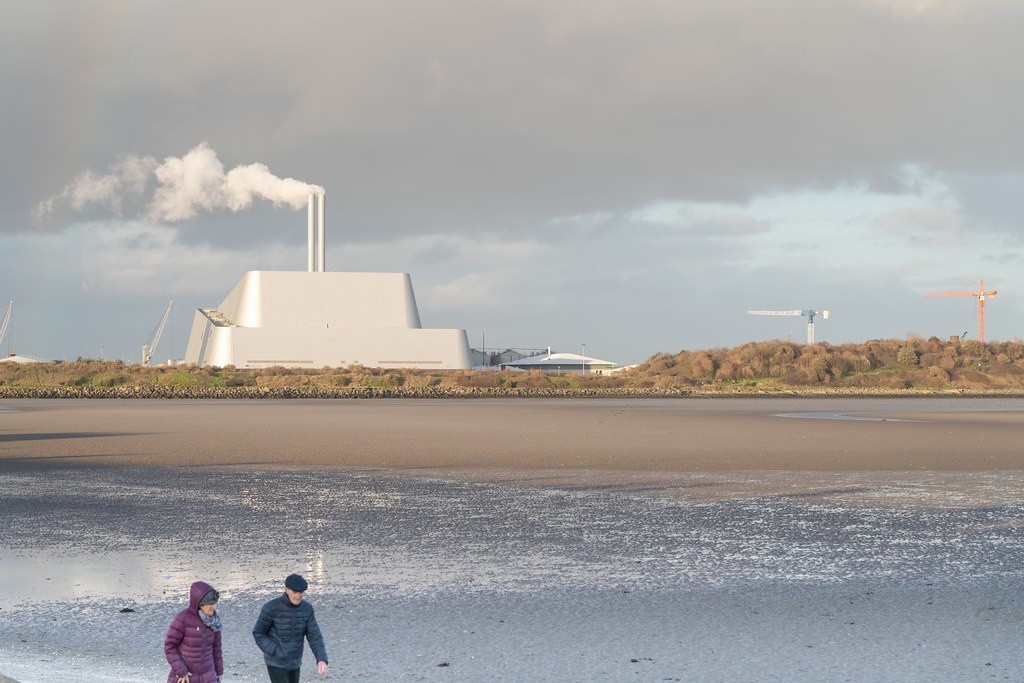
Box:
[252,574,328,683]
[164,581,224,683]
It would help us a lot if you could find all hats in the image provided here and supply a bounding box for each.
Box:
[285,574,308,593]
[199,590,219,606]
